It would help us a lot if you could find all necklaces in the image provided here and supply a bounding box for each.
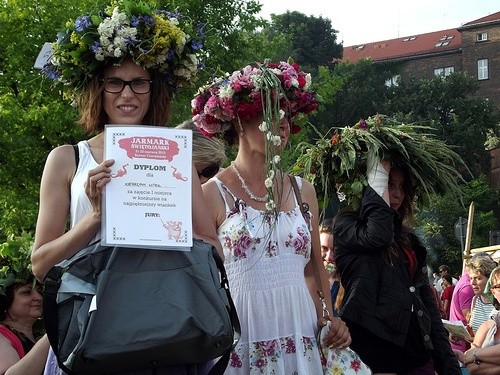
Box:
[231,161,278,201]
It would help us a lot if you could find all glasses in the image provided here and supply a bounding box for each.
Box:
[492,284,500,293]
[101,77,153,94]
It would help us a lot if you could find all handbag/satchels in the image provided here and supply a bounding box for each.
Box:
[36,239,241,375]
[317,316,371,375]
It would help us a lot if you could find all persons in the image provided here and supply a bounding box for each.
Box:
[201,81,351,375]
[31,35,225,375]
[0,272,50,375]
[333,143,462,375]
[319,218,345,317]
[426,252,500,375]
[176,121,226,185]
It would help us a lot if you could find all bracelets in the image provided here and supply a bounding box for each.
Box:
[473,348,480,365]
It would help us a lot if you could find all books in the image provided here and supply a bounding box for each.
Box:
[101,124,193,252]
